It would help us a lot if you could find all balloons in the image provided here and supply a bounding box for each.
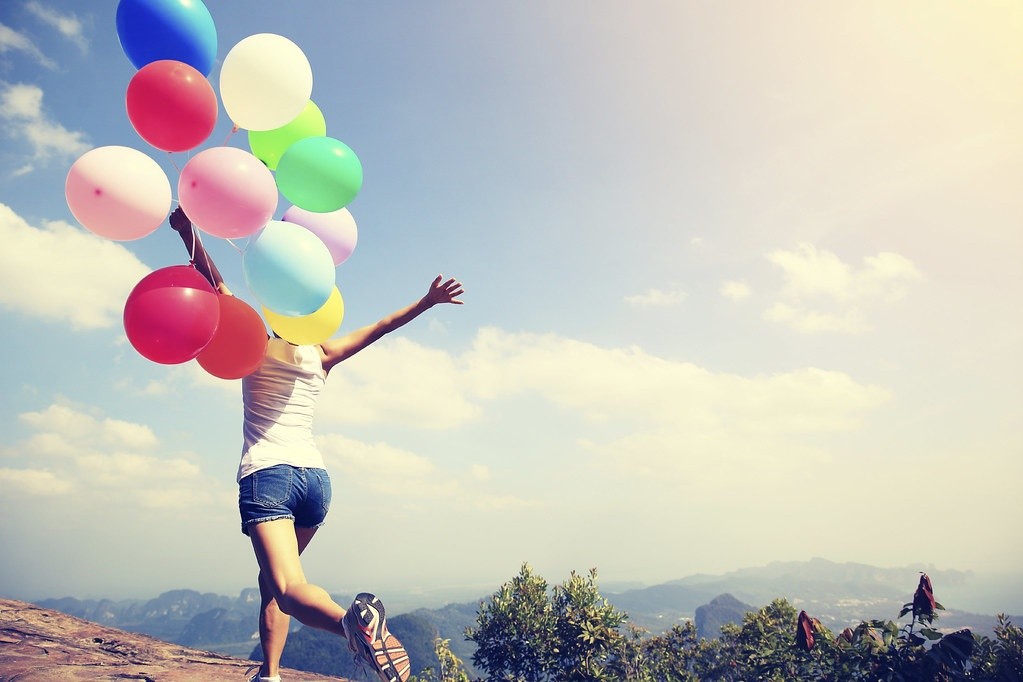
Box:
[123,260,219,364]
[276,136,363,214]
[248,99,326,171]
[115,0,217,78]
[126,60,218,153]
[261,285,344,345]
[220,33,313,131]
[282,205,358,266]
[196,294,268,381]
[242,221,336,317]
[178,147,278,240]
[65,146,173,242]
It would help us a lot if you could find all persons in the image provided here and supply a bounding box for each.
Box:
[169,204,465,682]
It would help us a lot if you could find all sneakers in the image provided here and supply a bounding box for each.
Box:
[245,664,282,682]
[343,593,410,682]
